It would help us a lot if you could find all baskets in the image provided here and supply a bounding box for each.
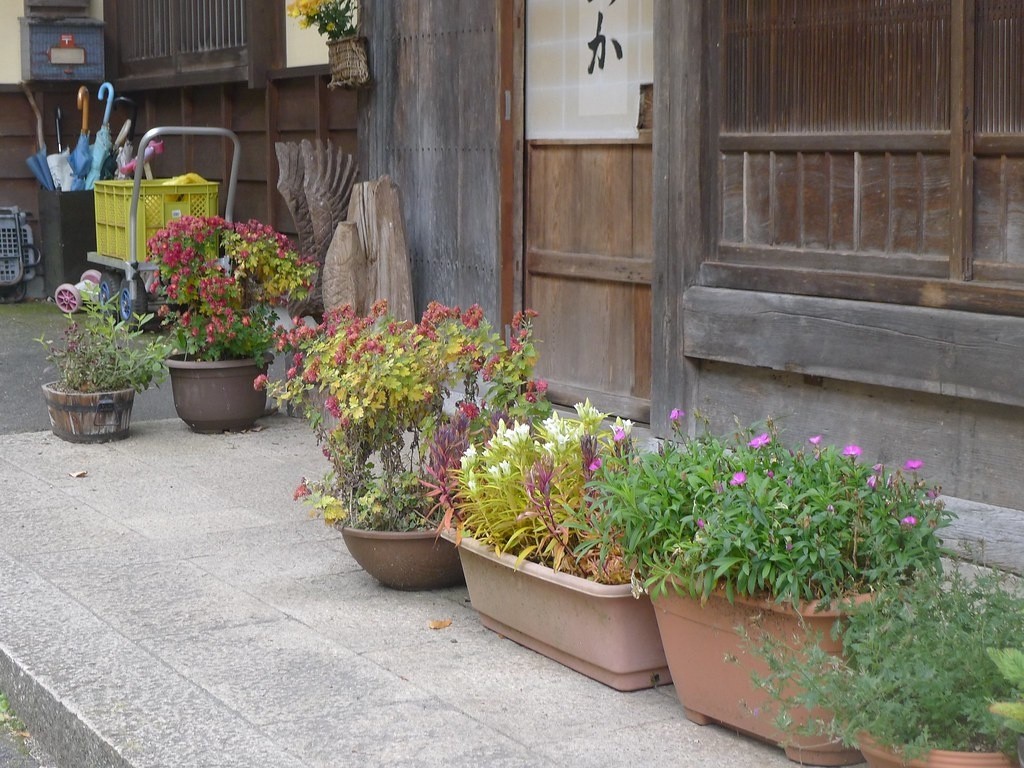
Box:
[326,34,369,87]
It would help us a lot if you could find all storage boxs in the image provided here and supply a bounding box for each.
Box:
[93,178,221,263]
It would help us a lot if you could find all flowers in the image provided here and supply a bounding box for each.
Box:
[577,408,960,610]
[30,281,174,393]
[143,214,321,368]
[406,397,643,584]
[287,0,359,39]
[724,538,1024,768]
[252,297,551,531]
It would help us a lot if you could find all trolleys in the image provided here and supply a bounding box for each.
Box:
[87,125,242,332]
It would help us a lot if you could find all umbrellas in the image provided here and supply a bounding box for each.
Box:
[26,81,137,192]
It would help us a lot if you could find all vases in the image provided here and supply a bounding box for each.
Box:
[163,348,275,435]
[41,381,136,444]
[439,527,673,692]
[333,524,466,590]
[629,558,918,768]
[841,718,1019,768]
[325,36,376,90]
